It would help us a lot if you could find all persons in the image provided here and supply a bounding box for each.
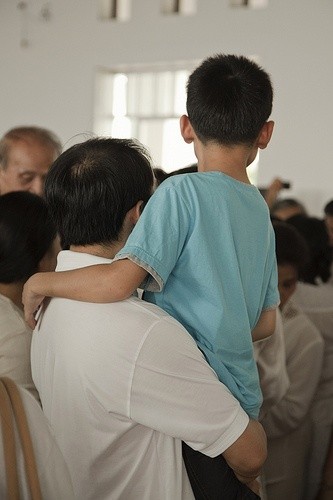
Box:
[249,221,325,500]
[269,196,307,221]
[289,212,333,500]
[19,55,279,499]
[31,140,268,499]
[0,189,62,410]
[0,126,63,198]
